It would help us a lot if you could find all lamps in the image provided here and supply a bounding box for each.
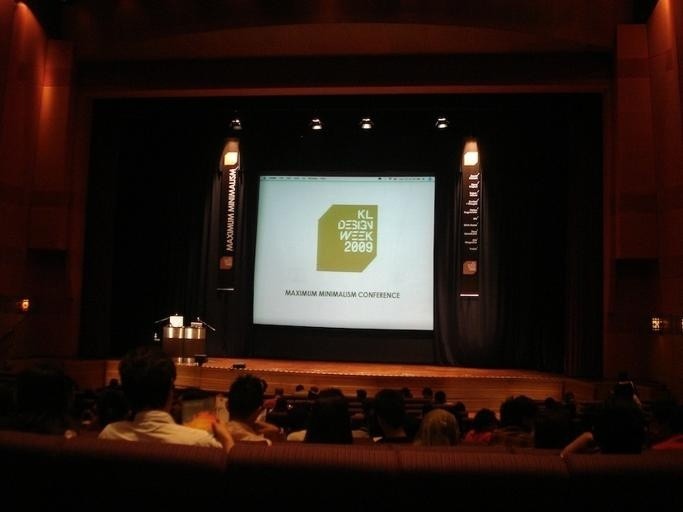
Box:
[14,295,31,314]
[645,310,664,336]
[226,109,453,137]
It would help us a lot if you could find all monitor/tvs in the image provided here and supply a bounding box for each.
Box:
[191,322,202,328]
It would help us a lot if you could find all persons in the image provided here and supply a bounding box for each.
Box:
[1,344,683,455]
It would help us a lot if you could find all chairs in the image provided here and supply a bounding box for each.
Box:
[0,373,683,512]
